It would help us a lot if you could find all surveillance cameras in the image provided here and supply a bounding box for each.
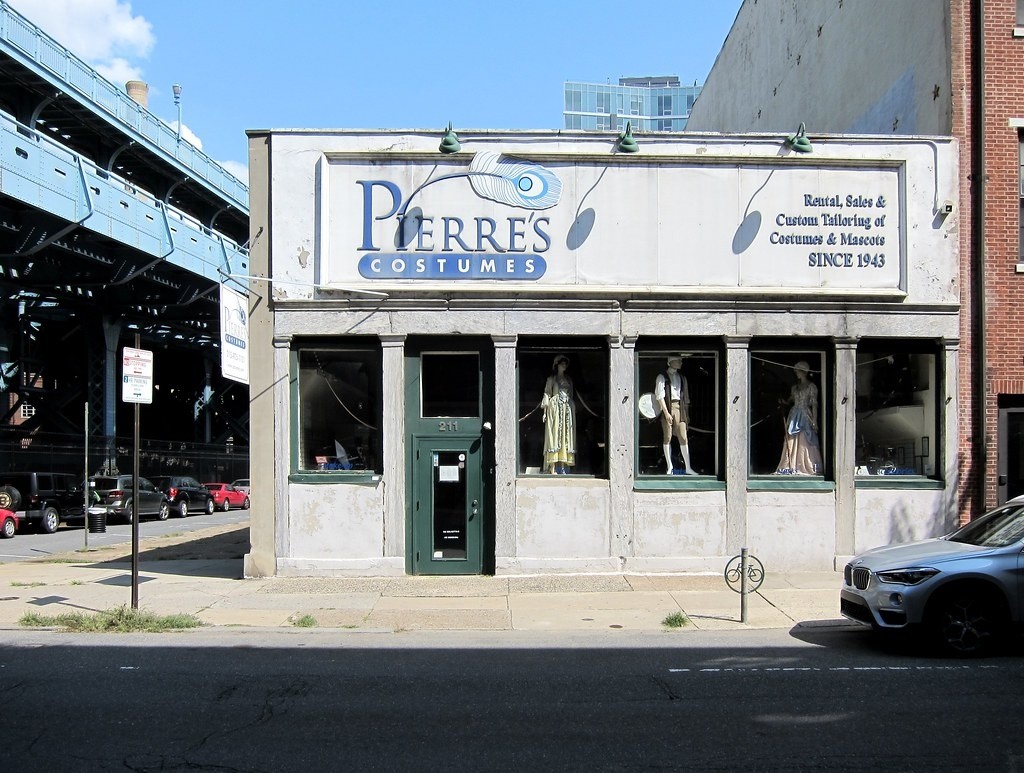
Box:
[942,205,952,214]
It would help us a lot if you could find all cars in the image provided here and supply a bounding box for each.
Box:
[0,509,20,539]
[838,494,1024,656]
[229,478,251,496]
[204,482,251,512]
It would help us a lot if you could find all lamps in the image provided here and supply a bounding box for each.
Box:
[439,121,460,152]
[620,120,639,153]
[785,122,813,153]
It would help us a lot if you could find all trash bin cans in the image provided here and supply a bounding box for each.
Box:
[88,508,108,533]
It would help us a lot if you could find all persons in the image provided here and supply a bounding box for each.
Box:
[654,355,700,476]
[776,362,823,476]
[541,356,576,474]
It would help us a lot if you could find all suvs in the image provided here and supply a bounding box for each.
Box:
[145,475,215,518]
[81,474,170,525]
[0,471,103,534]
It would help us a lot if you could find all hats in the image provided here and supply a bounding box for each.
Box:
[553,355,569,370]
[667,356,682,366]
[795,361,809,372]
